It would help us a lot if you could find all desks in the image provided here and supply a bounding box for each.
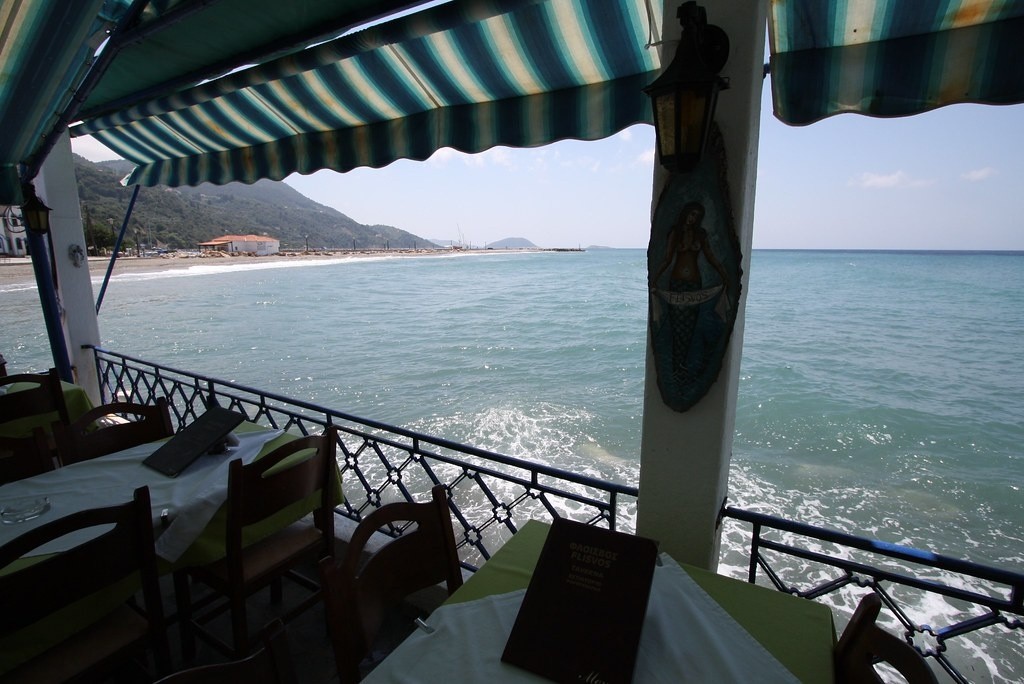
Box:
[0,421,346,675]
[360,519,838,684]
[0,374,98,457]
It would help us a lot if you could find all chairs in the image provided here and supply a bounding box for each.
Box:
[0,354,938,684]
